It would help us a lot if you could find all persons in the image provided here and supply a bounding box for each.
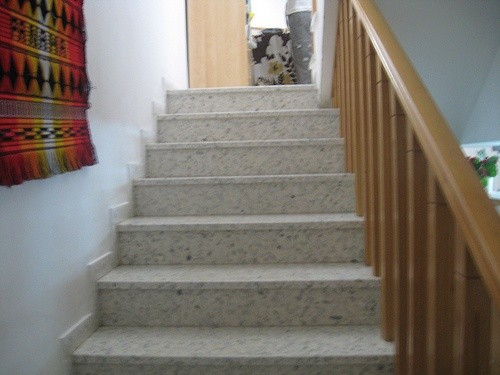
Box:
[285,0,319,83]
